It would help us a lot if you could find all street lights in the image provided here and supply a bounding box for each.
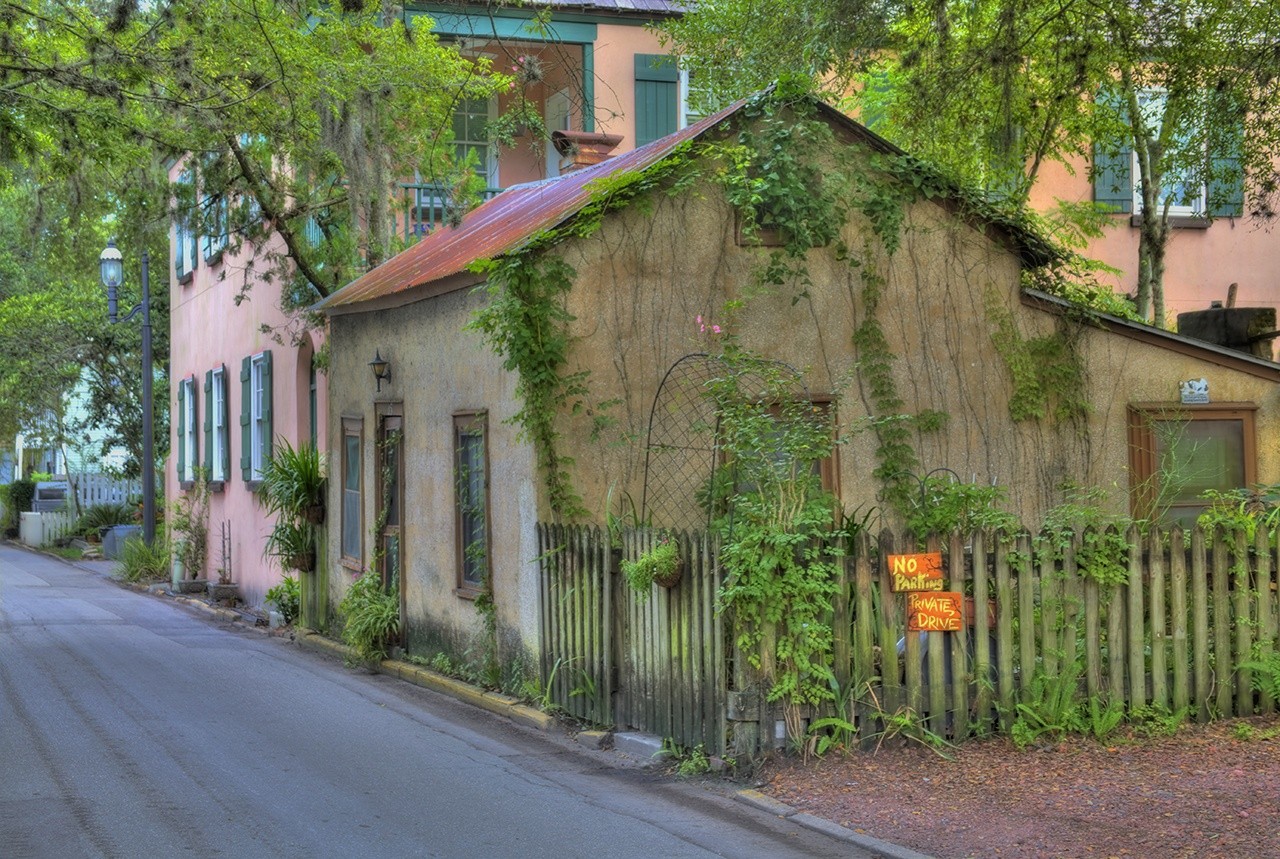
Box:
[99,235,156,548]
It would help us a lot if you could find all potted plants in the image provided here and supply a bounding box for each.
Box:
[205,520,239,599]
[943,576,999,630]
[259,513,316,573]
[252,434,326,524]
[615,527,683,608]
[168,463,206,593]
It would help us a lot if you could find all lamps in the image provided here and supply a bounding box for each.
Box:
[368,347,391,393]
